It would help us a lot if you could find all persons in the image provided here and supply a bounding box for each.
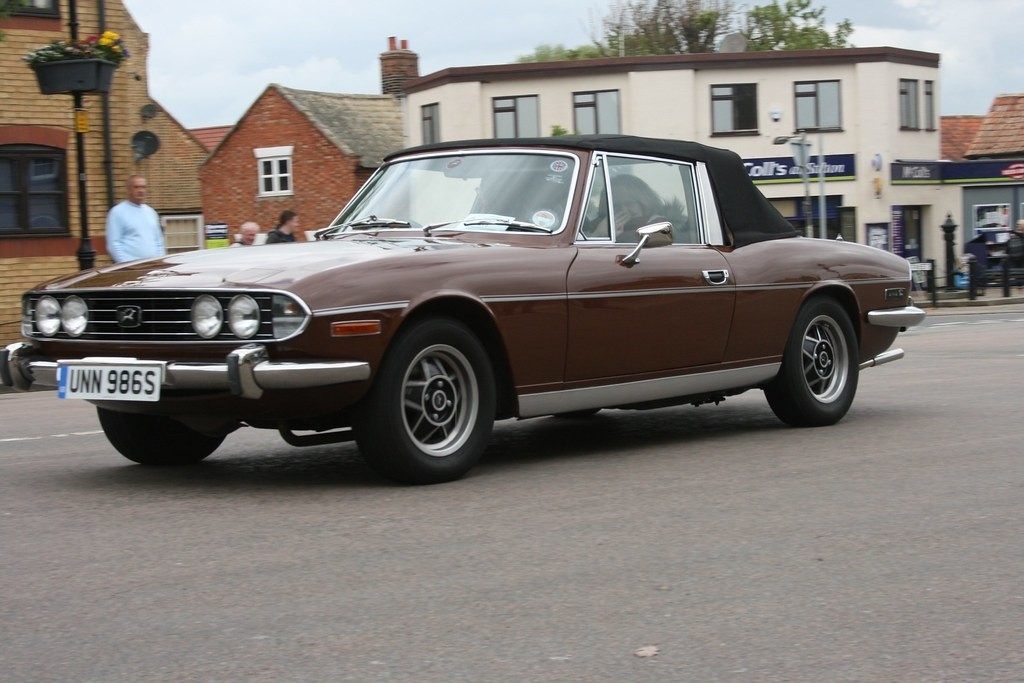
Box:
[105,175,165,263]
[477,172,526,222]
[587,174,689,242]
[265,210,299,245]
[1001,219,1024,286]
[231,222,259,248]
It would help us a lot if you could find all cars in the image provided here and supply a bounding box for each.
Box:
[0,132,929,484]
